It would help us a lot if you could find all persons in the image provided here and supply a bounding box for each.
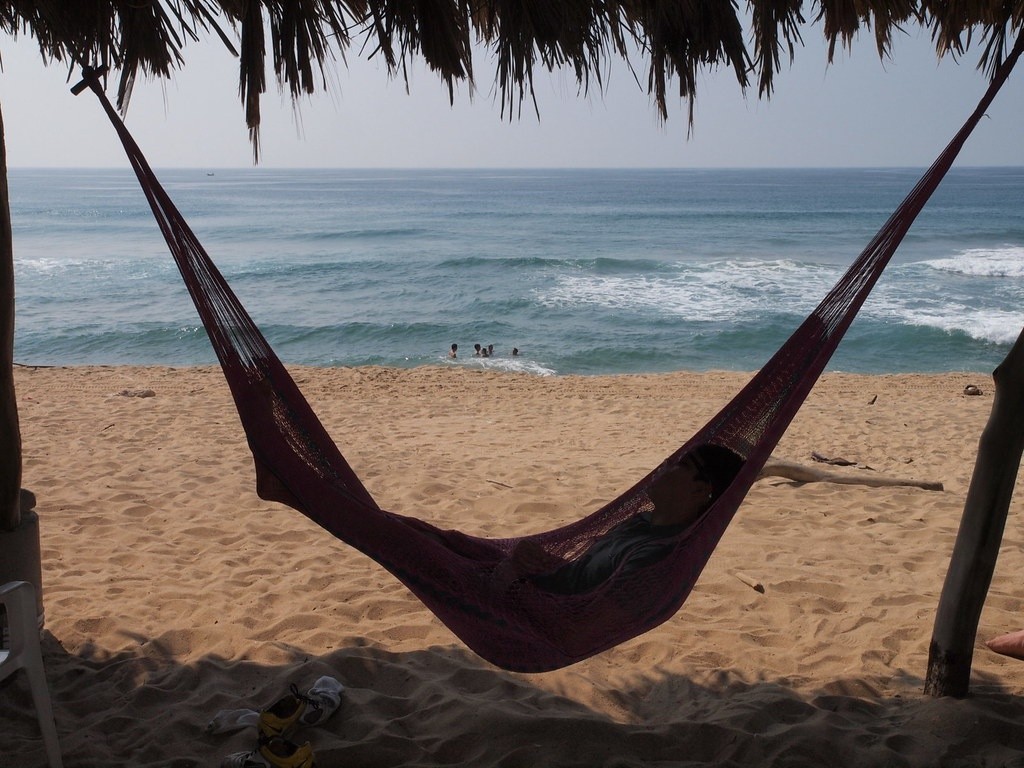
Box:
[449,344,457,358]
[488,345,494,355]
[245,380,745,673]
[512,348,518,355]
[481,348,489,357]
[474,344,481,356]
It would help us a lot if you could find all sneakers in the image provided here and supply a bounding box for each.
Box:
[221,735,318,768]
[256,682,341,745]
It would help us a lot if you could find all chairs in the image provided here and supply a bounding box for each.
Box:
[0,581,64,768]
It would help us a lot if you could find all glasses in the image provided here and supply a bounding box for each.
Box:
[678,452,709,484]
